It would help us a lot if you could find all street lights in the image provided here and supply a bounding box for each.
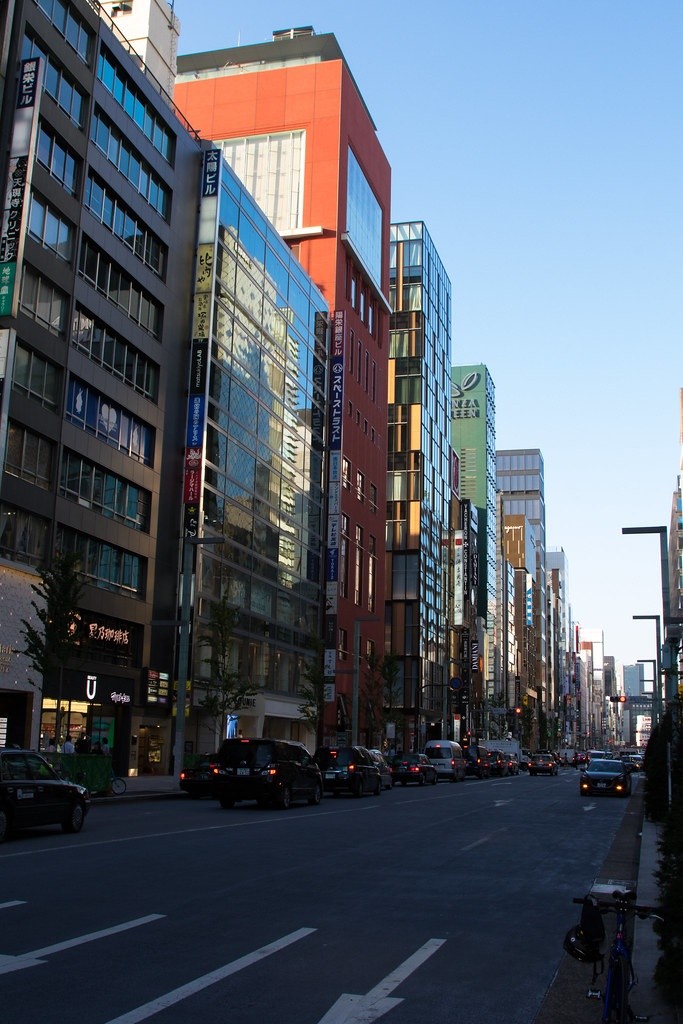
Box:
[631,614,664,724]
[171,536,226,794]
[622,524,669,716]
[636,657,658,730]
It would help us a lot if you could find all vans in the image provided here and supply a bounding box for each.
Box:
[213,737,324,811]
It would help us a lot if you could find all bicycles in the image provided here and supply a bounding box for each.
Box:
[574,889,665,1024]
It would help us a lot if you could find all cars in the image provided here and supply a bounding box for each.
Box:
[0,743,91,843]
[423,738,647,783]
[391,753,438,786]
[313,744,382,798]
[180,751,219,801]
[369,749,394,791]
[579,758,632,798]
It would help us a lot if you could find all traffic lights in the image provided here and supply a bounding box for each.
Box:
[610,696,627,703]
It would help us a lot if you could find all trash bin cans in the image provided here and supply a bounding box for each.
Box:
[38,751,114,791]
[184,752,203,769]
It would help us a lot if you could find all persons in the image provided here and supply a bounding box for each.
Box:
[47,732,109,755]
[563,753,569,770]
[381,746,403,756]
[237,729,242,739]
